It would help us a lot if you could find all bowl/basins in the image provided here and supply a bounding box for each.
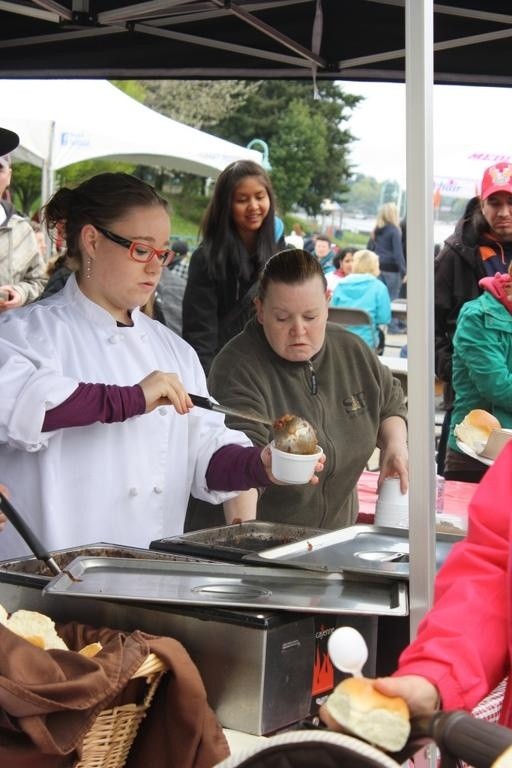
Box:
[269,440,325,486]
[1,540,381,737]
[148,518,465,586]
[372,476,410,531]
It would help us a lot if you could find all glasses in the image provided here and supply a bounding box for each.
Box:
[92,222,175,268]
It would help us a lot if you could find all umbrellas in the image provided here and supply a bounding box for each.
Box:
[1,79,273,230]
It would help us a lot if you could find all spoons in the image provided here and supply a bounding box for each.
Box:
[324,623,368,678]
[186,393,318,455]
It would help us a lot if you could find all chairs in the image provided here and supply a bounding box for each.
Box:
[390,298,407,322]
[327,307,377,356]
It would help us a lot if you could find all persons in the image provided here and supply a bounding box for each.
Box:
[322,437,511,742]
[286,222,305,250]
[305,231,320,251]
[445,268,512,484]
[182,160,282,380]
[0,150,48,311]
[169,242,191,278]
[325,248,358,304]
[434,163,512,478]
[330,250,391,357]
[186,247,409,536]
[367,202,408,302]
[310,237,337,275]
[0,483,13,533]
[0,171,326,561]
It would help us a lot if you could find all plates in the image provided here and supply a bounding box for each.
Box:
[455,427,512,467]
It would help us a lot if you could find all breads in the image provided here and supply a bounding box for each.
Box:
[319,677,411,753]
[6,608,69,650]
[0,602,7,622]
[453,408,501,449]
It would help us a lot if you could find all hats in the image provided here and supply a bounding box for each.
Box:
[1,127,20,159]
[480,162,511,201]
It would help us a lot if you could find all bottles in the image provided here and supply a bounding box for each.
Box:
[436,471,444,514]
